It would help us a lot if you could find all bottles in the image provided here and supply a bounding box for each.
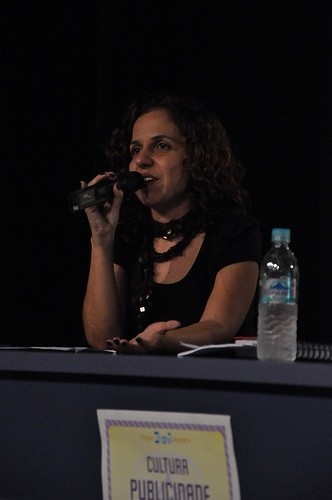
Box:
[256,229,298,361]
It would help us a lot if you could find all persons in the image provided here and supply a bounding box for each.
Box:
[80,94,260,353]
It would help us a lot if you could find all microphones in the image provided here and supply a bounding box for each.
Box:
[70,171,146,209]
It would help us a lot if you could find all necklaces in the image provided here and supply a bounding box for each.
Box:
[144,210,203,264]
